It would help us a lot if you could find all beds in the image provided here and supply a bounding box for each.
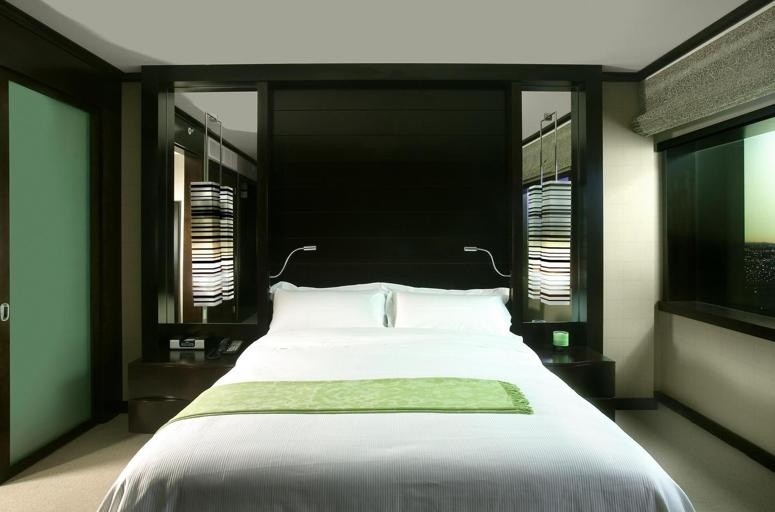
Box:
[96,282,696,511]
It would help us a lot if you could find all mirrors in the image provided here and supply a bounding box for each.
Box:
[173,83,258,325]
[521,85,586,323]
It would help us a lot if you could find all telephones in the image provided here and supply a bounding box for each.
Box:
[217,337,245,354]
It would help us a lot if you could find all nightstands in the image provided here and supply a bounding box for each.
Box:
[127,344,238,435]
[523,343,615,422]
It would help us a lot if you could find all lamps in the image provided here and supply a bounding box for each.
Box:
[270,245,320,278]
[526,121,542,299]
[218,118,235,300]
[190,111,223,309]
[540,110,573,305]
[463,246,511,278]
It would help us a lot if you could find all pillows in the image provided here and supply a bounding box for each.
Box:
[269,282,386,328]
[382,284,514,331]
